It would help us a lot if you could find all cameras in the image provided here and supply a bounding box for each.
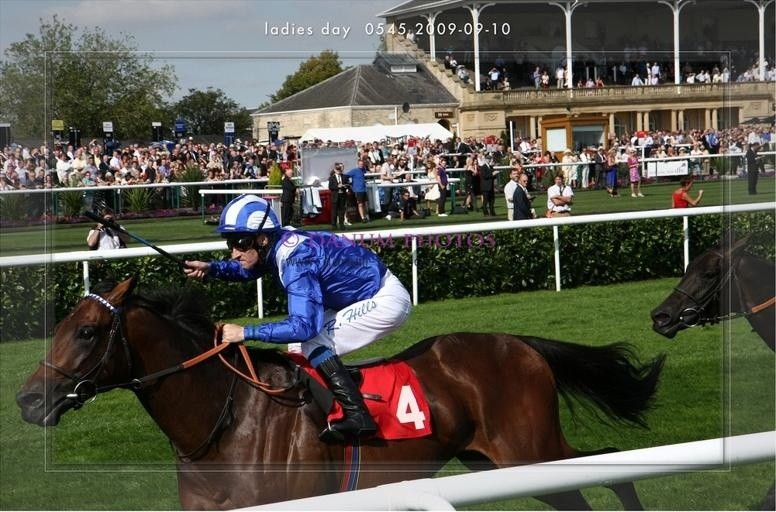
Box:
[105,217,115,223]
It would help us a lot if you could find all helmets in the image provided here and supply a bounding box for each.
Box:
[217,194,280,233]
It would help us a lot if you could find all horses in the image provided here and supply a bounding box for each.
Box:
[14,270,671,512]
[647,231,776,511]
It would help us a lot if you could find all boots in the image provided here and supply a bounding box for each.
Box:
[316,355,378,442]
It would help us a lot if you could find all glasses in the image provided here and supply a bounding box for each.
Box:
[227,236,255,251]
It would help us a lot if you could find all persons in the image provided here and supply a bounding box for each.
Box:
[444,54,511,91]
[184,193,412,446]
[86,208,130,250]
[405,29,415,43]
[533,56,775,92]
[1,125,775,228]
